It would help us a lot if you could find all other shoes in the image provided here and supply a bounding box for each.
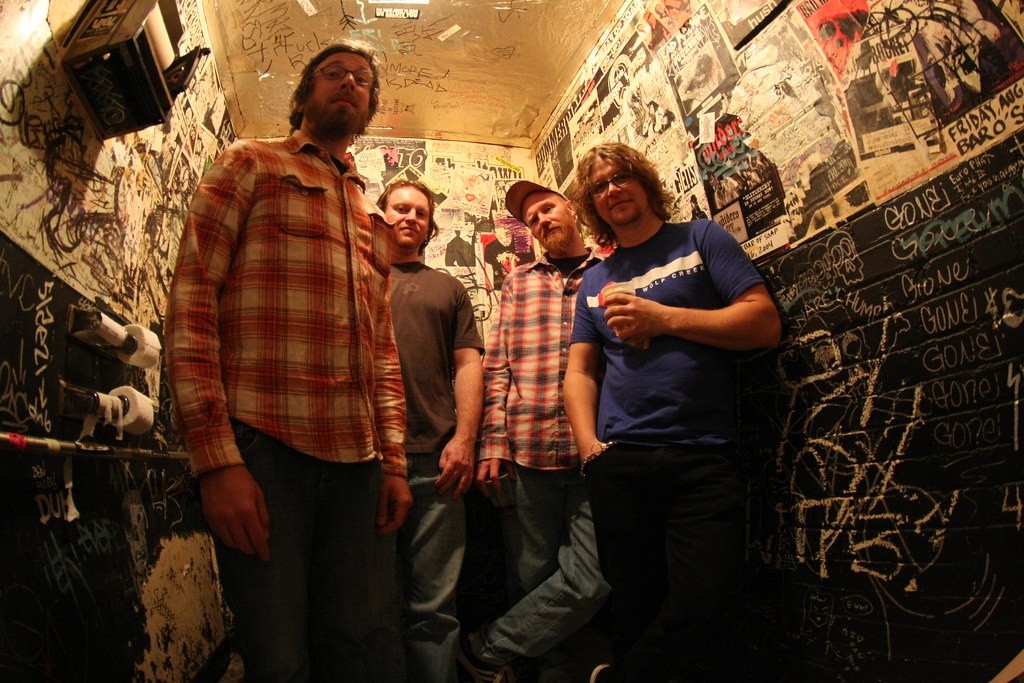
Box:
[588,663,612,683]
[451,633,519,683]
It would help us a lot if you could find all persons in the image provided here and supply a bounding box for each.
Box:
[164,44,413,683]
[563,142,781,683]
[473,181,611,683]
[377,180,484,683]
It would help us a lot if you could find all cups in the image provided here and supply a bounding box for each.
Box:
[601,281,636,338]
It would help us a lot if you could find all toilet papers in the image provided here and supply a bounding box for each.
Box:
[116,324,162,368]
[107,386,154,434]
[147,2,175,71]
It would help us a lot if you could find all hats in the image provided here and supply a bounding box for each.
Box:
[506,180,569,227]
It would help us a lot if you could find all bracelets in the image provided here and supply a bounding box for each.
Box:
[580,441,614,477]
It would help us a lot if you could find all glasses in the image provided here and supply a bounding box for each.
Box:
[589,169,636,197]
[313,62,374,89]
